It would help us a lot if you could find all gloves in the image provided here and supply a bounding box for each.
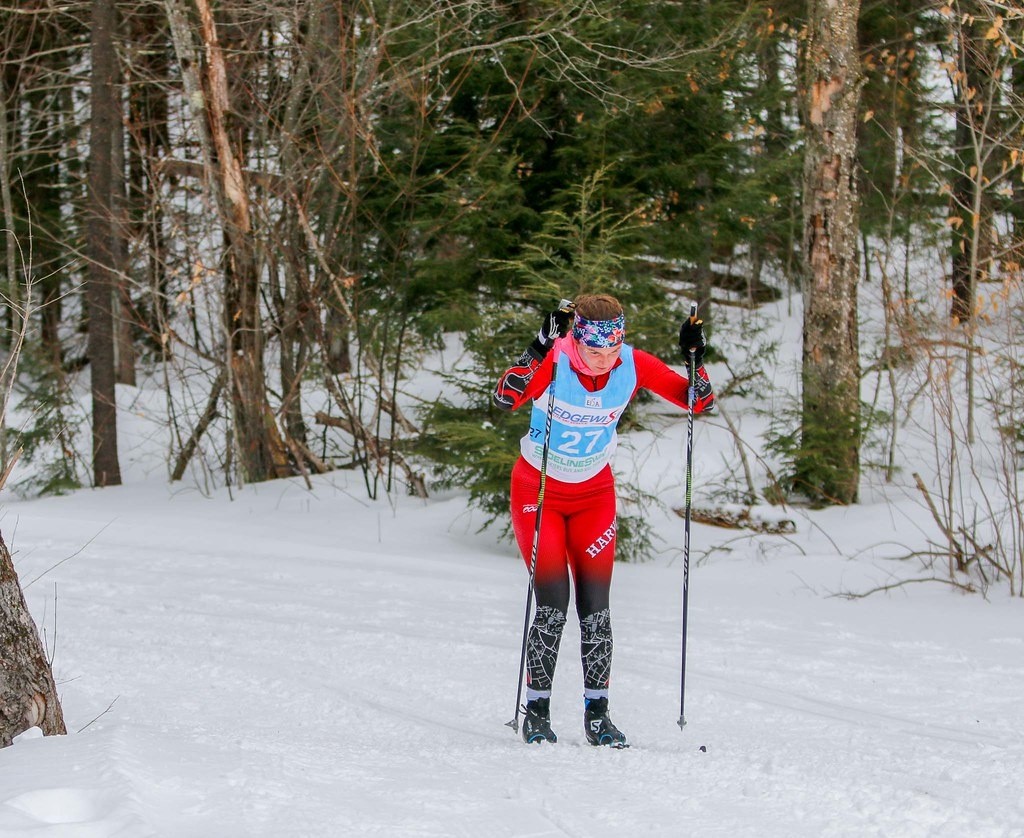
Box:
[530,309,574,357]
[679,315,707,368]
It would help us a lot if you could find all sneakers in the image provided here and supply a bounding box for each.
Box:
[585,710,626,748]
[518,704,557,745]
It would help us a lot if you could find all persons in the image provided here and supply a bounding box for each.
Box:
[493,294,714,748]
